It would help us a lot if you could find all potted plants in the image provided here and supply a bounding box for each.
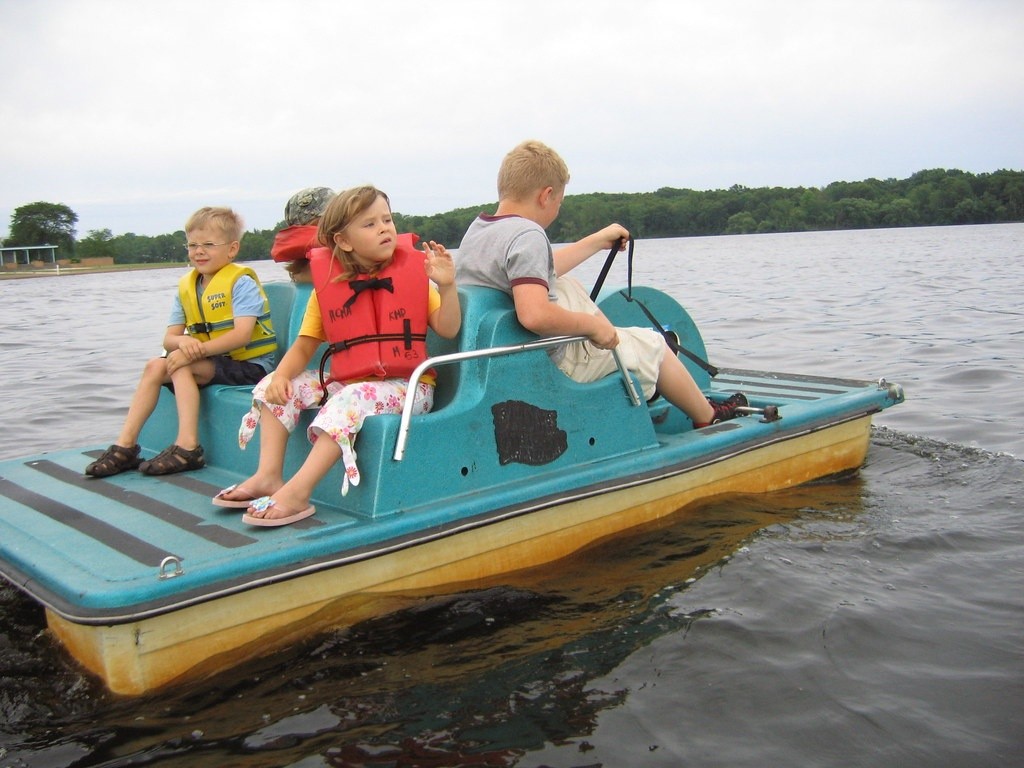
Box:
[68,258,85,267]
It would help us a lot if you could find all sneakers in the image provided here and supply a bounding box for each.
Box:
[644,331,681,403]
[691,393,751,429]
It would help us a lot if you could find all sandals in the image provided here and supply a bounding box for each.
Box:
[138,443,207,474]
[84,444,146,478]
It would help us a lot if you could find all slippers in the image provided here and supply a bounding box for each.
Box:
[242,496,317,527]
[211,483,256,508]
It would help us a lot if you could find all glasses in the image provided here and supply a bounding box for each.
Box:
[182,241,227,252]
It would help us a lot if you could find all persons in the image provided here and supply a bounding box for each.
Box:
[452,138,749,429]
[85,205,280,478]
[270,186,336,280]
[212,185,461,526]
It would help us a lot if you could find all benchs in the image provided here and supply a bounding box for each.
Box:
[484,285,660,463]
[137,280,474,521]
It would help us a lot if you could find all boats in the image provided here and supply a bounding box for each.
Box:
[0,232,904,710]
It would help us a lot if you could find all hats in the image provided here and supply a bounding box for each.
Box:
[284,185,339,226]
